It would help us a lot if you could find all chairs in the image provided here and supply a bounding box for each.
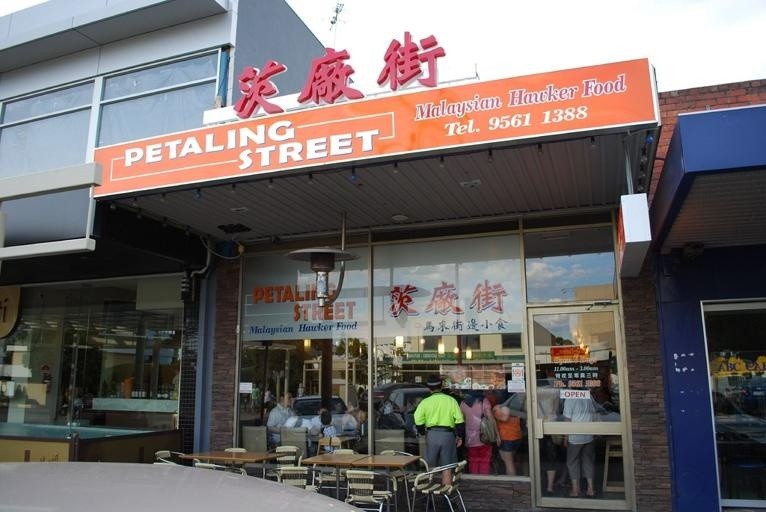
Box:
[153,437,467,512]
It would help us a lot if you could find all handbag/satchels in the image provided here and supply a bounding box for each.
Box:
[479,401,497,445]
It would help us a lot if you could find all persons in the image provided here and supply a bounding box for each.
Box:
[413,374,465,510]
[250,383,273,417]
[535,369,620,498]
[381,374,527,478]
[264,392,367,454]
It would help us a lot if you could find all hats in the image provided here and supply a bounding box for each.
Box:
[425,375,442,388]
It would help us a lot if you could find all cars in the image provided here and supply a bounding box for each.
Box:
[264,372,623,454]
[708,376,765,479]
